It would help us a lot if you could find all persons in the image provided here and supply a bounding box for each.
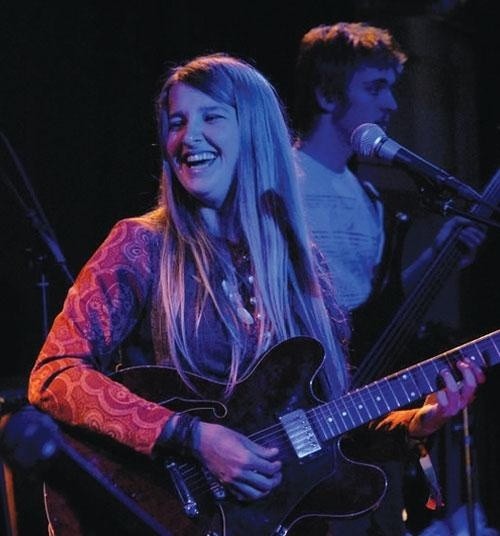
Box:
[287,20,487,352]
[27,52,487,505]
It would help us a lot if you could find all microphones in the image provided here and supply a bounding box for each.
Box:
[349,122,484,206]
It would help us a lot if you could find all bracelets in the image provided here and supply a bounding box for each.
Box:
[169,414,201,457]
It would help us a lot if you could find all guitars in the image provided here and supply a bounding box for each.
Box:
[348,169,498,391]
[42,331,499,535]
